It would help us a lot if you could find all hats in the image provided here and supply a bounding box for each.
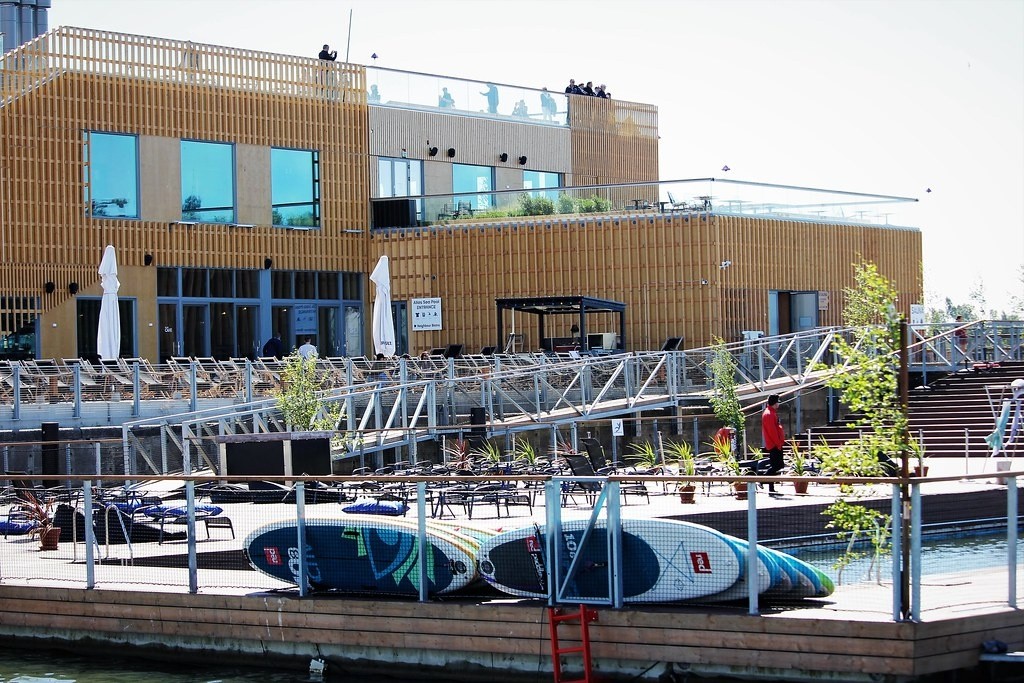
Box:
[378,354,385,358]
[403,354,412,360]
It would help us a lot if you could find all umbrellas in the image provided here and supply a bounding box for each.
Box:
[97,245,121,359]
[369,256,396,357]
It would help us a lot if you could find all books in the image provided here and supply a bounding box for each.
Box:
[565,79,611,125]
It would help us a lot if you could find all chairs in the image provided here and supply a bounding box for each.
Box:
[2,436,665,546]
[438,200,473,221]
[667,190,686,212]
[1,337,687,406]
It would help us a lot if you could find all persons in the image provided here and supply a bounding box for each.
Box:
[366,353,416,382]
[540,87,557,121]
[319,45,337,96]
[512,99,529,119]
[368,85,379,101]
[955,316,969,369]
[263,332,284,369]
[756,394,784,497]
[297,336,318,369]
[439,87,456,108]
[479,83,499,113]
[177,40,199,79]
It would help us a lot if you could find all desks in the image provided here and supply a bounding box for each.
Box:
[625,189,896,226]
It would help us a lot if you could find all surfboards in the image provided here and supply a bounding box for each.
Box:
[241,514,501,595]
[477,519,835,605]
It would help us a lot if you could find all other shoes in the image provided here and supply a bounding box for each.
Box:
[758,484,764,489]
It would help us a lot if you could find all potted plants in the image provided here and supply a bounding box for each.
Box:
[439,437,854,505]
[908,431,929,477]
